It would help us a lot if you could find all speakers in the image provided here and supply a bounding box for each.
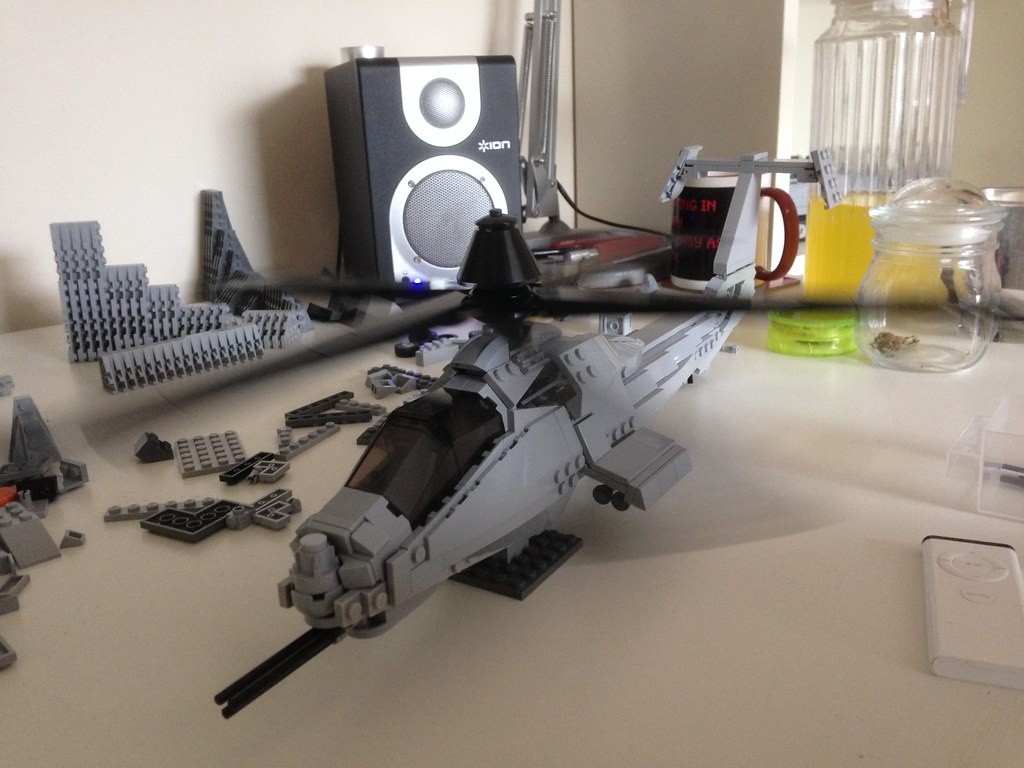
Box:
[325,53,520,295]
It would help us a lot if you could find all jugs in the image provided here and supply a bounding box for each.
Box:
[805,0,973,214]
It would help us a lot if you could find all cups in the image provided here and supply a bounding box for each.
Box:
[670,171,798,290]
[855,178,1009,373]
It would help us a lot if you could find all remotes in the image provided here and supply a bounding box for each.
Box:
[922,535,1024,690]
